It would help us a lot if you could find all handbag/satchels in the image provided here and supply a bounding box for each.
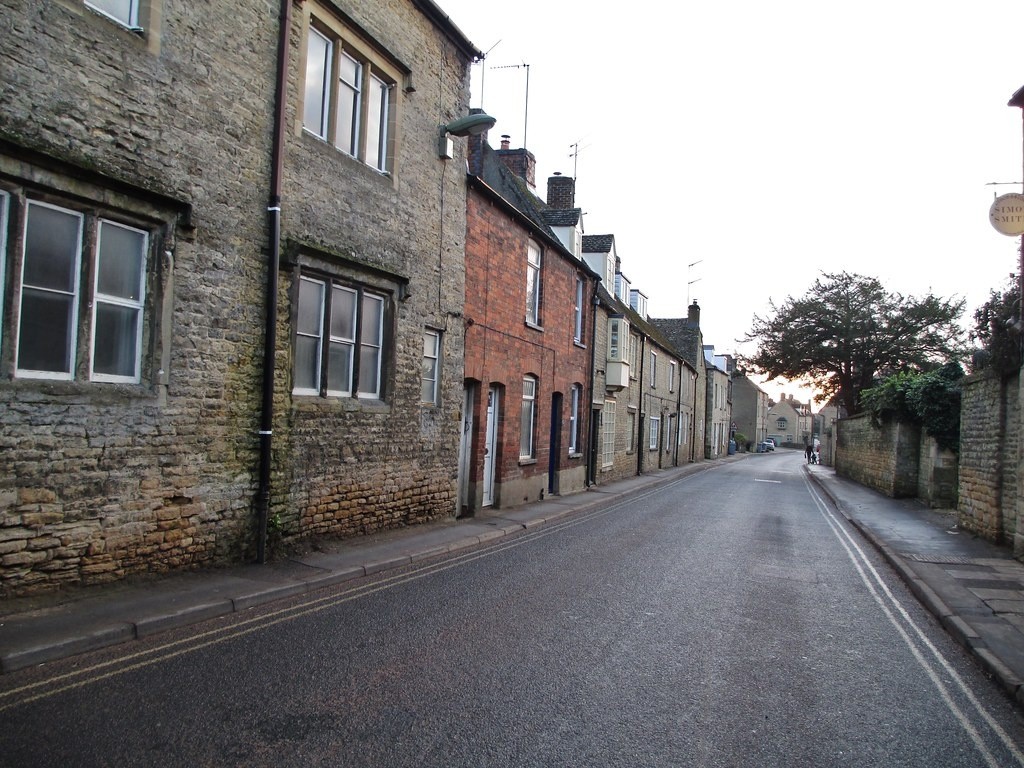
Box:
[805,453,806,458]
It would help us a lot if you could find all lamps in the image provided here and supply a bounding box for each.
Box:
[438,114,497,137]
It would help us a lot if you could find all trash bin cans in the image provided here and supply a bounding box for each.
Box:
[757,443,762,453]
[762,444,767,452]
[728,440,736,455]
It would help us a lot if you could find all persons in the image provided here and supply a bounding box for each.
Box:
[805,444,814,464]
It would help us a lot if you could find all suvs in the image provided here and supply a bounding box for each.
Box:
[764,439,774,451]
